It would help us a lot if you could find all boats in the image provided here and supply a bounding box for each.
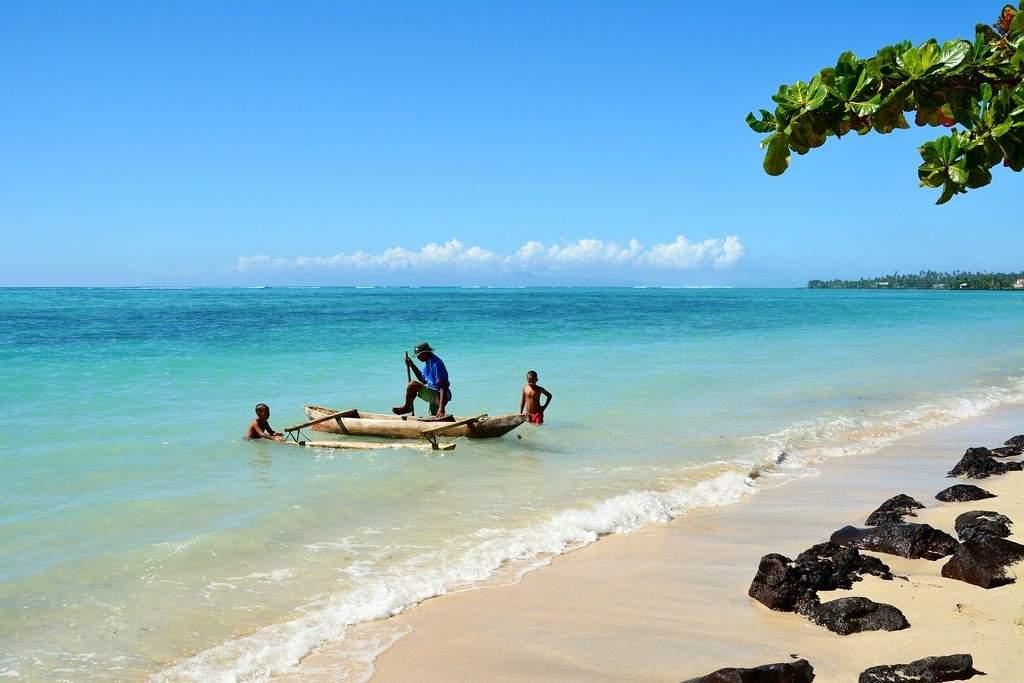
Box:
[282,404,530,451]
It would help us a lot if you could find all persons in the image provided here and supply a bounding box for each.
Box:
[245,403,283,442]
[519,371,552,424]
[392,343,452,418]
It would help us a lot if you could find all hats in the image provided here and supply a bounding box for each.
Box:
[410,342,435,357]
